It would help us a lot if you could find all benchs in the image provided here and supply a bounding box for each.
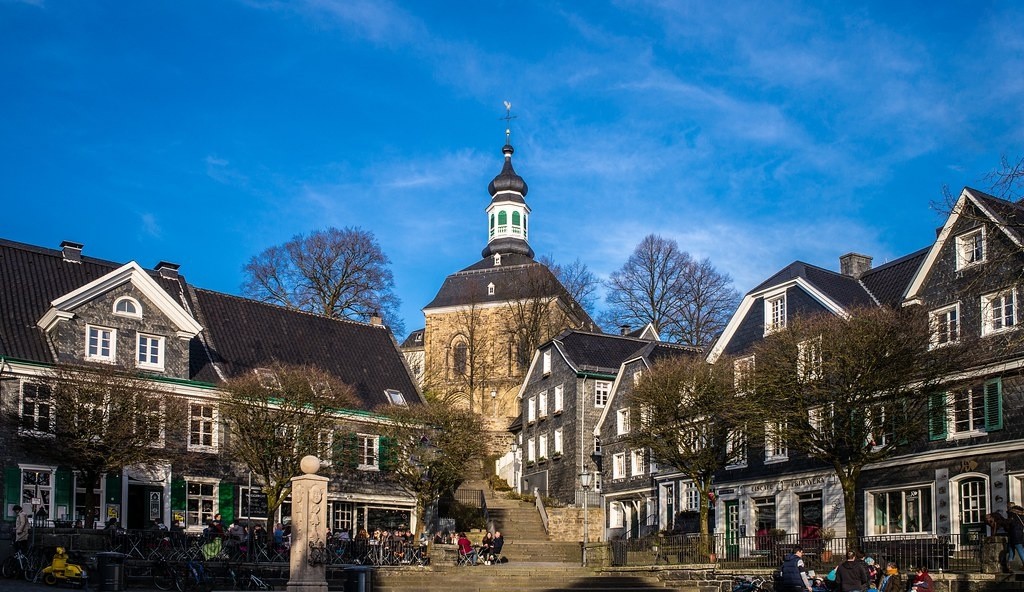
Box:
[781,552,816,567]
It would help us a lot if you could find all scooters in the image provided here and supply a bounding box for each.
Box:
[41,547,89,588]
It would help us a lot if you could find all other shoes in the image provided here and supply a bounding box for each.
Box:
[488,561,491,565]
[484,561,487,565]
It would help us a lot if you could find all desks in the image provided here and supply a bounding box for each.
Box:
[47,519,78,528]
[471,546,487,564]
[778,548,817,567]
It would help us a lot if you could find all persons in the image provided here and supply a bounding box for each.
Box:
[826,548,883,592]
[874,561,902,592]
[1006,502,1024,567]
[73,513,292,564]
[778,548,812,592]
[907,566,936,592]
[12,504,30,568]
[326,526,504,567]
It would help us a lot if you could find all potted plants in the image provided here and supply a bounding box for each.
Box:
[819,527,836,563]
[537,456,546,464]
[526,460,535,468]
[551,451,562,460]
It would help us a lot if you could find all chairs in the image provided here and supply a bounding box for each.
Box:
[98,529,502,566]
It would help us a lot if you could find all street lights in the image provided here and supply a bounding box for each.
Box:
[32,473,46,544]
[246,469,257,563]
[510,441,518,492]
[577,464,594,567]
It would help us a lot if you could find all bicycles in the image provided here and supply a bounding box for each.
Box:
[732,575,771,592]
[152,555,275,592]
[1,533,43,582]
